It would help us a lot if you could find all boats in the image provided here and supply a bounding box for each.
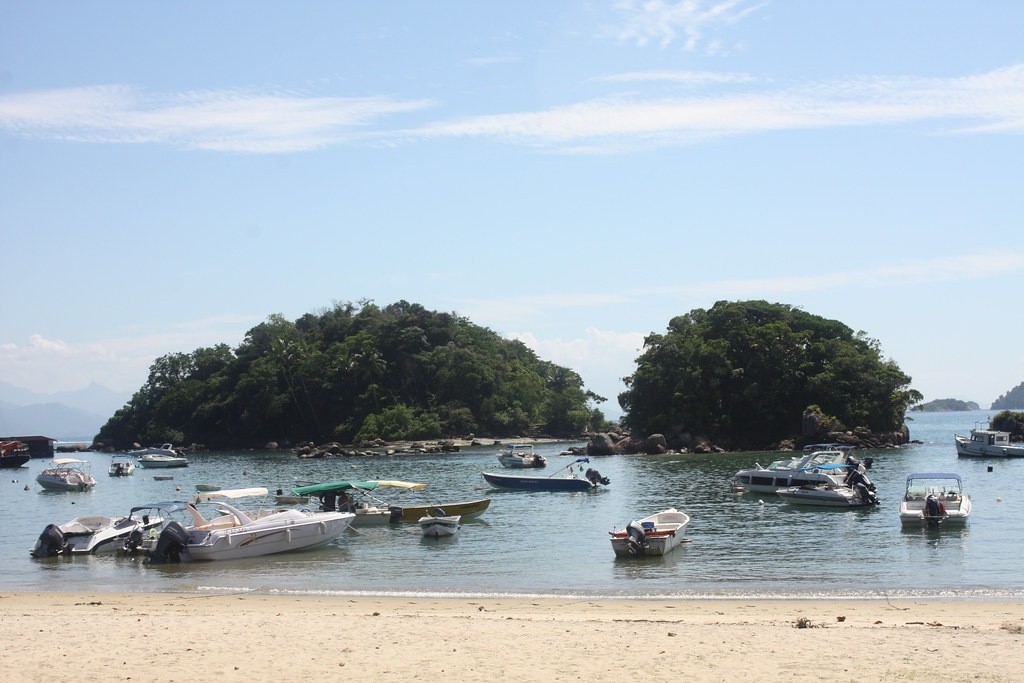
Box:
[482,458,593,491]
[36,459,97,489]
[137,444,188,467]
[735,444,877,505]
[418,509,461,535]
[954,422,1024,456]
[126,487,356,559]
[496,445,546,467]
[898,473,971,525]
[609,508,690,555]
[367,481,491,516]
[29,515,164,554]
[0,441,30,466]
[292,483,401,523]
[275,489,310,503]
[108,455,135,476]
